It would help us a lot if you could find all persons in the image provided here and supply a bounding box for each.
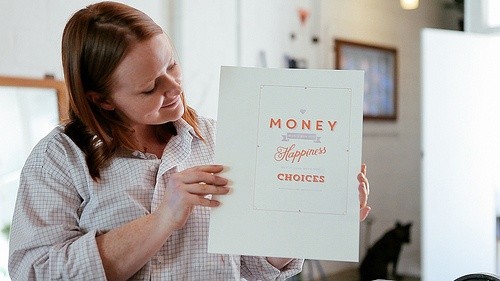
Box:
[7,1,371,281]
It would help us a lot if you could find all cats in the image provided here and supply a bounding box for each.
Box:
[360,221,412,281]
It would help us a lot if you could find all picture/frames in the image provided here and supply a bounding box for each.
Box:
[334,39,399,119]
